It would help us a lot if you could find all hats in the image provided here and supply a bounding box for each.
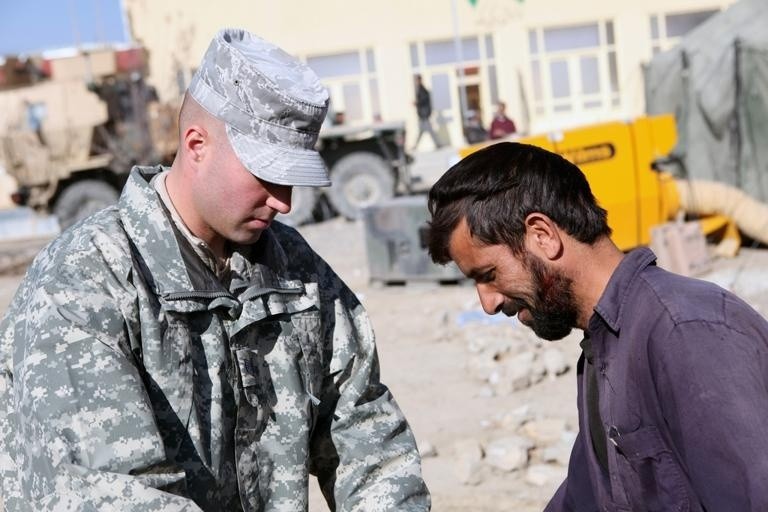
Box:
[190,28,332,187]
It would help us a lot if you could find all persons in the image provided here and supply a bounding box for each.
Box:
[485,102,524,140]
[0,27,432,512]
[428,144,767,512]
[403,75,448,149]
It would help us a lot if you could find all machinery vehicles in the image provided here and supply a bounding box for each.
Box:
[0,69,411,241]
